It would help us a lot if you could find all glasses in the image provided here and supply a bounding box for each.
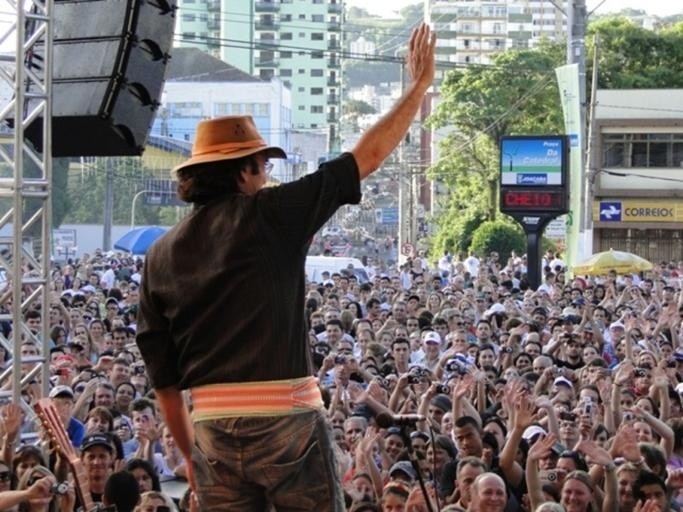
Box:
[264,160,274,175]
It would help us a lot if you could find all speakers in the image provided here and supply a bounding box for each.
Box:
[4,0,177,156]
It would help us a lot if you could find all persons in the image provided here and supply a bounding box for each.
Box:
[136,22,436,512]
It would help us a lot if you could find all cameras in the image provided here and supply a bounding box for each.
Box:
[377,378,391,386]
[502,345,514,353]
[335,356,346,363]
[641,362,651,369]
[416,369,427,377]
[600,369,612,376]
[447,363,459,371]
[135,366,144,373]
[407,376,420,384]
[623,411,633,421]
[538,471,557,484]
[49,482,68,495]
[436,385,450,394]
[634,368,648,377]
[554,368,565,376]
[663,359,676,368]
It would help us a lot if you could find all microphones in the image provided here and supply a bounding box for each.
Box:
[376,412,426,428]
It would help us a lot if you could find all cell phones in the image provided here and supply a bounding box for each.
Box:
[636,491,646,507]
[560,412,576,421]
[583,403,592,425]
[141,414,151,431]
[53,369,68,375]
[120,418,127,430]
[581,397,591,414]
[563,333,577,338]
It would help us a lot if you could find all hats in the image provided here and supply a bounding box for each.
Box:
[48,384,75,399]
[424,331,441,345]
[521,425,548,440]
[609,322,625,331]
[169,114,288,175]
[388,460,418,480]
[554,375,573,388]
[79,433,113,451]
